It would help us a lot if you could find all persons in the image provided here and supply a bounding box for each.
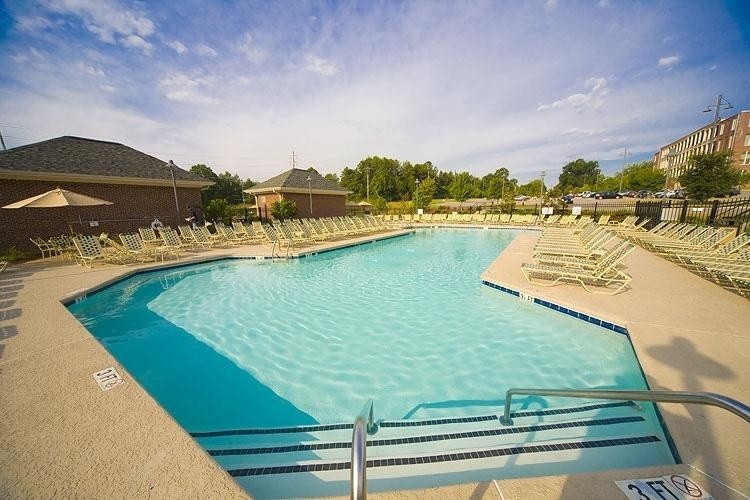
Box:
[151,217,163,233]
[185,205,199,231]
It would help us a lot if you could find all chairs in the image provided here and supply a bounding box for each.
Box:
[30,213,473,270]
[473,214,750,295]
[0,261,8,273]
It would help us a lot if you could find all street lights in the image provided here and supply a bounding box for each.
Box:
[364,166,371,203]
[415,180,420,208]
[501,173,506,199]
[307,175,313,216]
[539,170,546,221]
[662,148,679,206]
[165,160,180,215]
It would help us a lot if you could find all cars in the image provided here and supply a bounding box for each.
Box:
[722,186,740,198]
[577,189,689,199]
[561,196,574,204]
[515,194,530,201]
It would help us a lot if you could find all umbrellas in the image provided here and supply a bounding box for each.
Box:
[356,200,374,208]
[1,183,114,236]
[346,200,359,216]
